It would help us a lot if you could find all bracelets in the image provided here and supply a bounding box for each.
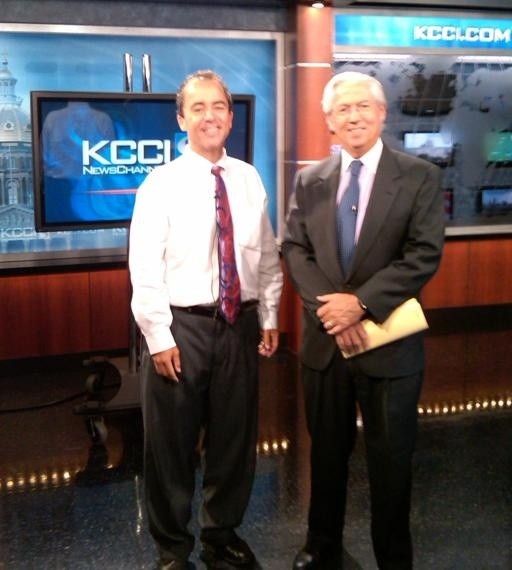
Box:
[359,298,368,311]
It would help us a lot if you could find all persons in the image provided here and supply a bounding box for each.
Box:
[280,71,446,569]
[126,68,285,568]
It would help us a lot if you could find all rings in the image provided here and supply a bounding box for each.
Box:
[328,319,334,328]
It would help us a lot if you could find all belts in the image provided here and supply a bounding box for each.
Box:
[170,298,260,316]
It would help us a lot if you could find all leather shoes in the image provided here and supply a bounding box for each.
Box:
[291,548,342,570]
[154,557,189,570]
[199,527,255,564]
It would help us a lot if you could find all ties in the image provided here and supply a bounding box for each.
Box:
[336,160,363,278]
[210,167,242,326]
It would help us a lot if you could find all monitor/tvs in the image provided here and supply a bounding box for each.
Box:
[401,128,456,168]
[483,128,512,168]
[29,88,256,234]
[478,185,512,217]
